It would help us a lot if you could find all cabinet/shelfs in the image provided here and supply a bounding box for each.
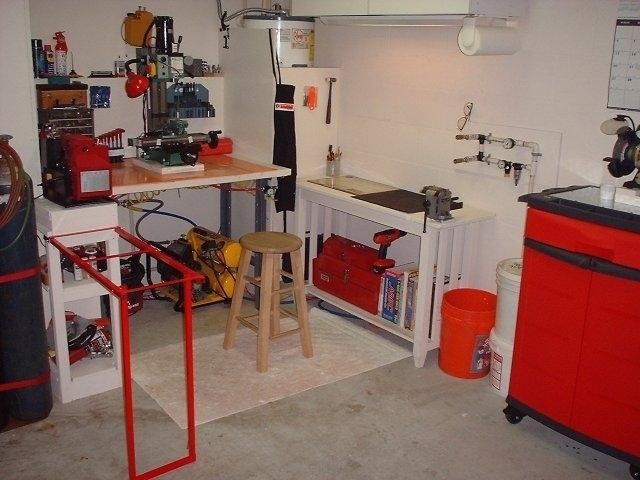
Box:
[500,184,640,479]
[288,1,525,19]
[45,219,124,406]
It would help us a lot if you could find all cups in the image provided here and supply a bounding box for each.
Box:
[324,178,341,189]
[599,200,616,210]
[325,158,341,177]
[599,184,617,201]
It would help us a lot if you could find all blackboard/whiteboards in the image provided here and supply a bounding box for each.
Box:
[607,17,640,111]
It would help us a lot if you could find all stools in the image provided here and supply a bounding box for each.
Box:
[220,229,316,376]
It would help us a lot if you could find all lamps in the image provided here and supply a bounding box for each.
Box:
[121,55,155,100]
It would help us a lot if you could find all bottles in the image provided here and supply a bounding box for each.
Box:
[72,256,98,282]
[31,38,55,79]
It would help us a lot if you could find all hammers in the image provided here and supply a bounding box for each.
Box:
[325,78,336,123]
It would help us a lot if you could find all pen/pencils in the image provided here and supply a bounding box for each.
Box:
[327,145,342,176]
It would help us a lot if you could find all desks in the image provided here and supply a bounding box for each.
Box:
[293,172,497,370]
[111,149,293,313]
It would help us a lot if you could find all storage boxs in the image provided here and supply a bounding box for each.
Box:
[311,233,396,317]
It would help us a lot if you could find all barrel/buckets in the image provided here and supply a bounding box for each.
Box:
[489,257,523,398]
[438,289,497,379]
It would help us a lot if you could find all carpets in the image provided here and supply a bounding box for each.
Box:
[121,290,414,433]
